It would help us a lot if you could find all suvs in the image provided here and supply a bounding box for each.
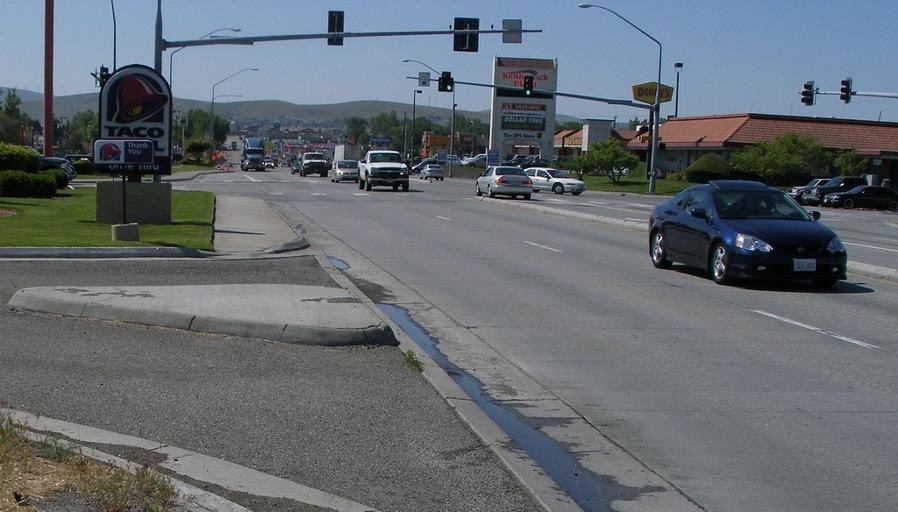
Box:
[786,176,897,214]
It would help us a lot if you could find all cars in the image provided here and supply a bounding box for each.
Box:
[523,166,585,197]
[474,165,533,200]
[231,136,408,193]
[647,176,849,288]
[24,146,93,189]
[405,152,565,179]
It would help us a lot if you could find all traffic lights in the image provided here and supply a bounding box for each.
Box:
[839,77,852,104]
[99,66,109,88]
[523,75,533,96]
[440,71,452,91]
[799,80,814,106]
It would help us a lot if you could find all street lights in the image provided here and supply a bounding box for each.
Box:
[168,26,242,87]
[411,88,423,163]
[210,66,258,160]
[402,58,455,177]
[673,61,683,117]
[578,2,662,195]
